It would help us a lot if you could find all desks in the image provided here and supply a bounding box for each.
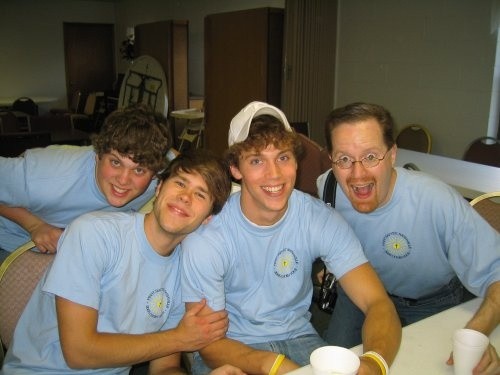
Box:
[281,295,500,375]
[393,148,500,201]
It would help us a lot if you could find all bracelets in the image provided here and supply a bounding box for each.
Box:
[361,351,389,375]
[270,353,287,375]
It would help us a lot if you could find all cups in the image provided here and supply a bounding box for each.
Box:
[310,345,361,375]
[452,328,490,375]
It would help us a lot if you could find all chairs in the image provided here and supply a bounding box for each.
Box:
[395,123,432,154]
[0,94,104,158]
[173,107,204,153]
[462,137,500,168]
[469,192,500,235]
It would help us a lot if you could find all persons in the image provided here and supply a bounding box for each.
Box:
[182,101,401,375]
[0,147,230,375]
[316,103,500,375]
[1,104,175,266]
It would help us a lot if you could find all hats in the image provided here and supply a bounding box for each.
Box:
[227,101,292,148]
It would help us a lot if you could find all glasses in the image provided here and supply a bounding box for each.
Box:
[329,146,391,169]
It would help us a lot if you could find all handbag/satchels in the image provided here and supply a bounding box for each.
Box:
[319,170,337,314]
